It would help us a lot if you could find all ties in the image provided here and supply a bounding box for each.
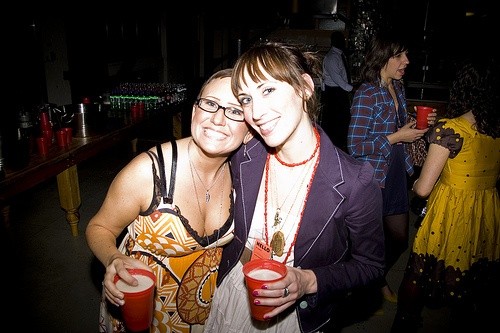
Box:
[341,53,351,85]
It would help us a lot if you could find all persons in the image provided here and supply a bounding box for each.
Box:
[321,31,356,146]
[391,60,500,333]
[346,33,437,316]
[203,35,383,333]
[86,68,256,333]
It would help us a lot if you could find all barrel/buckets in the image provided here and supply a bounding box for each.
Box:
[56,103,110,137]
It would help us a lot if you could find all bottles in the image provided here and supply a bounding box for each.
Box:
[111,80,188,111]
[15,111,40,154]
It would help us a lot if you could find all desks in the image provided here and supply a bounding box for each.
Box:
[0,100,183,238]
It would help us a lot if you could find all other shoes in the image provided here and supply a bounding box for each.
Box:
[381,285,398,304]
[364,294,385,316]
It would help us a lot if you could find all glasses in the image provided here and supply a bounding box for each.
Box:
[196,98,245,122]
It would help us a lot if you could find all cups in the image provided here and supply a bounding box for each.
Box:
[56,127,73,147]
[416,106,433,130]
[35,112,54,154]
[114,270,157,332]
[128,102,145,121]
[243,259,288,322]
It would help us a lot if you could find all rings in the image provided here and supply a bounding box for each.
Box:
[284,287,289,297]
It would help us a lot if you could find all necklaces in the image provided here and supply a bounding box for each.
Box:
[264,128,321,264]
[189,140,227,255]
